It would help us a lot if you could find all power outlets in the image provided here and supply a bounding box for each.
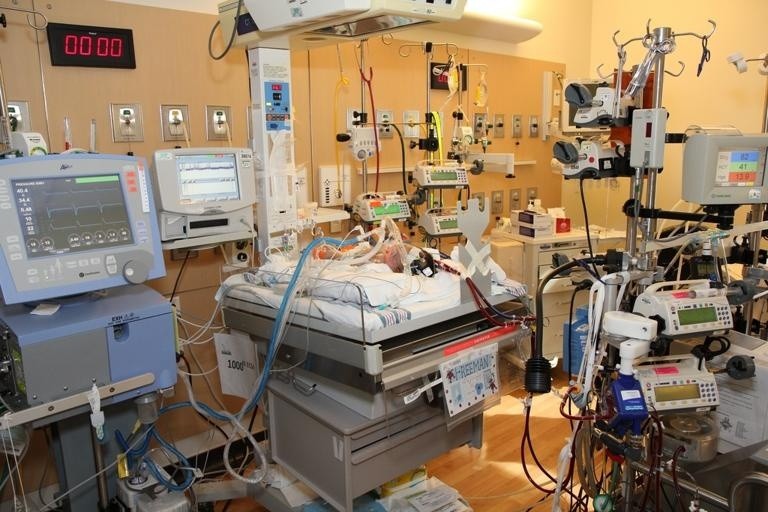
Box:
[473,114,486,138]
[403,111,419,138]
[113,107,144,143]
[3,103,30,141]
[493,114,505,138]
[377,112,393,137]
[159,105,193,142]
[204,104,233,141]
[346,109,361,129]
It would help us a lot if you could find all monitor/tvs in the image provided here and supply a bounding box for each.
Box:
[0,153,167,307]
[150,146,257,217]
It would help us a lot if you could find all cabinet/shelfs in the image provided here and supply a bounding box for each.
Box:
[479,225,602,365]
